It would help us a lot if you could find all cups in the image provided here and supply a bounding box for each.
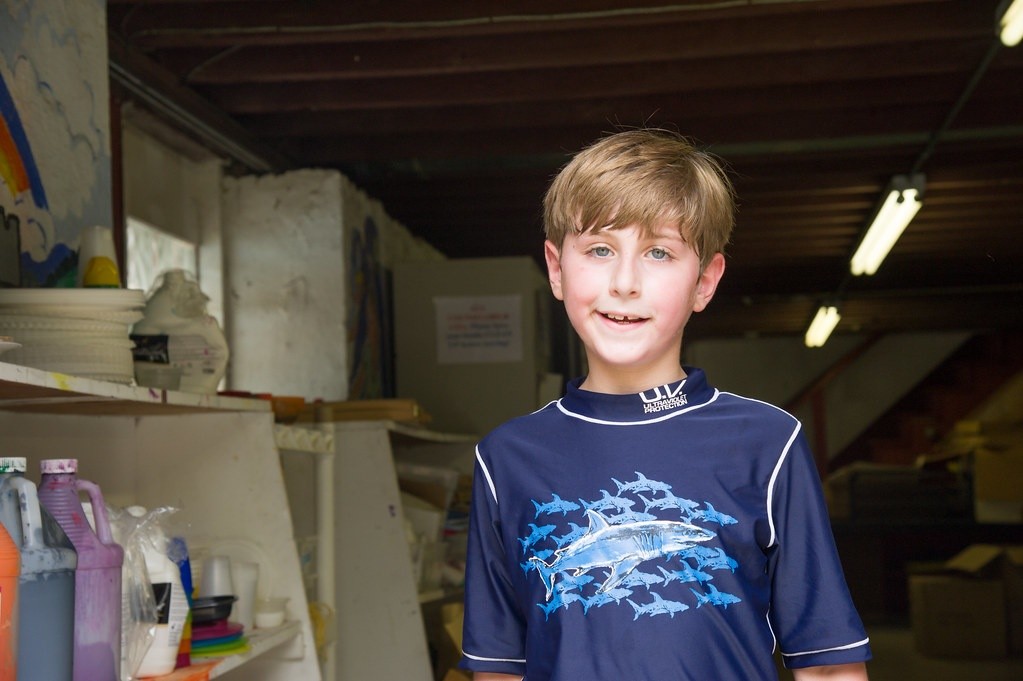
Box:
[164,536,287,670]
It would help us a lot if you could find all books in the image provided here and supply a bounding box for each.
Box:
[0,287,149,387]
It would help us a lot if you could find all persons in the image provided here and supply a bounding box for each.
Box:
[453,123,874,681]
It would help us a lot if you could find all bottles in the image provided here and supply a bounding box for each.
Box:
[0,523,22,680]
[1,456,81,680]
[30,456,127,680]
[112,505,187,678]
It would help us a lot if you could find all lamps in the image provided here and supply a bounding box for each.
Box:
[804,302,841,349]
[848,173,927,279]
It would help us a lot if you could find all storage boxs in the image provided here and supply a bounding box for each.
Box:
[307,399,431,422]
[908,571,1009,659]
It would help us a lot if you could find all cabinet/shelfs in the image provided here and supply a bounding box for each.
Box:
[278,420,485,681]
[0,349,336,681]
